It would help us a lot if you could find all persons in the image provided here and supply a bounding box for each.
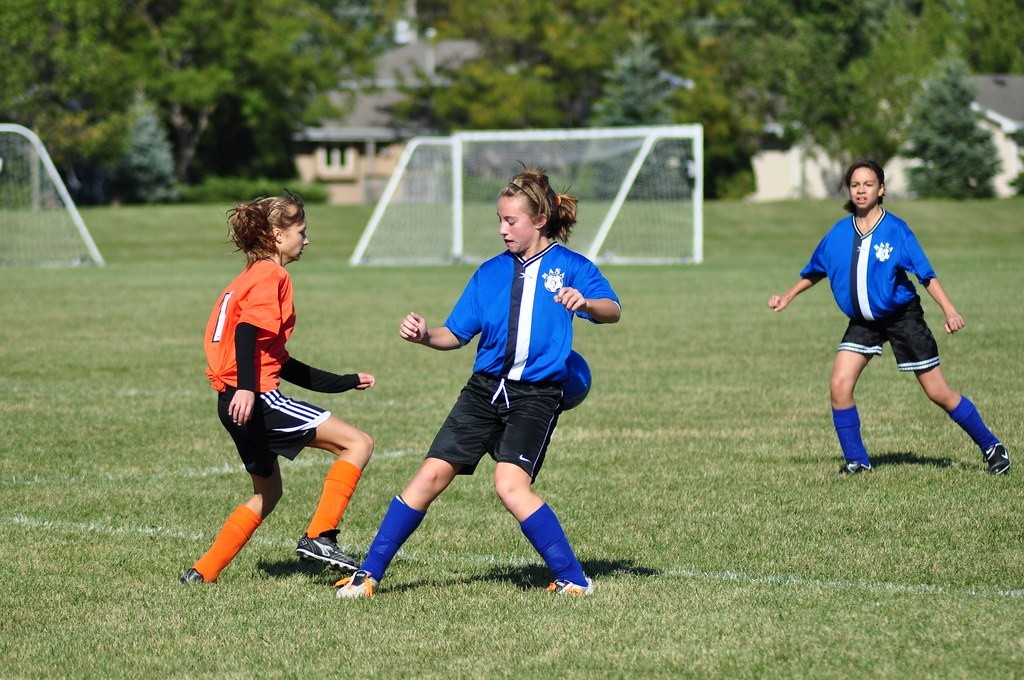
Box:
[336,160,621,600]
[179,190,375,590]
[769,161,1010,476]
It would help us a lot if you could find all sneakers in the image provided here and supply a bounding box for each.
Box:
[546,577,594,597]
[295,529,359,573]
[331,570,380,600]
[837,461,873,475]
[179,568,217,587]
[982,443,1011,474]
